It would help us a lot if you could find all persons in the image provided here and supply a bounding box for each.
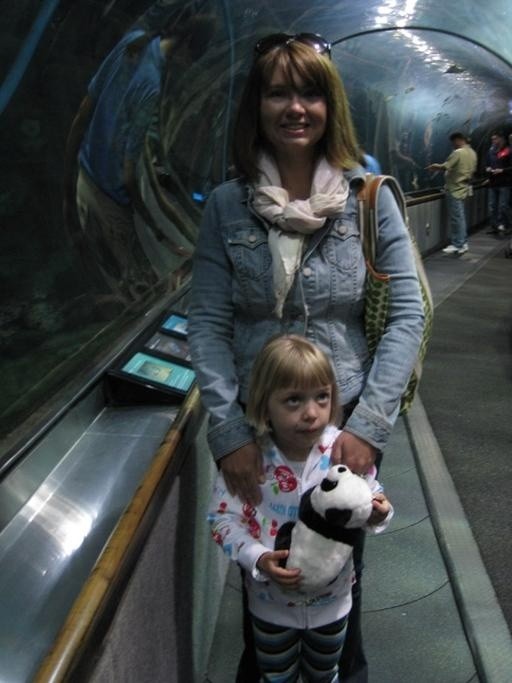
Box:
[423,131,512,255]
[356,146,382,176]
[205,335,394,682]
[61,12,218,312]
[184,32,426,680]
[390,133,416,179]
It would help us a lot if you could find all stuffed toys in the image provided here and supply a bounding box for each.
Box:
[274,466,383,594]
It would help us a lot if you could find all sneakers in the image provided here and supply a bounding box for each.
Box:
[448,242,468,250]
[442,245,465,253]
[498,224,506,231]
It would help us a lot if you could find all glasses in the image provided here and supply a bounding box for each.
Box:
[254,32,332,60]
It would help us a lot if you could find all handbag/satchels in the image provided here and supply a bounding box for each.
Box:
[356,171,434,415]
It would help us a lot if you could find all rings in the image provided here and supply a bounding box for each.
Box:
[357,472,369,479]
